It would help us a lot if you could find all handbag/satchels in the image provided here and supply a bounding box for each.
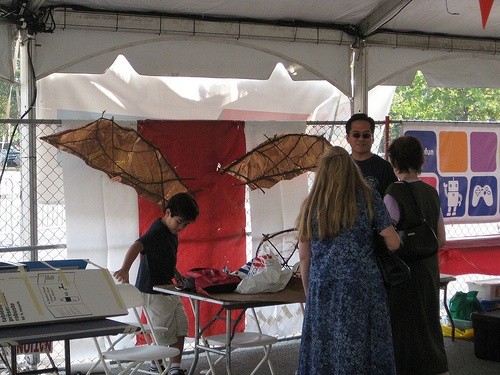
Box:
[365,193,411,286]
[184,267,241,295]
[233,252,293,295]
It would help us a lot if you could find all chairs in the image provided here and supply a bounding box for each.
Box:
[86,284,179,375]
[189,298,278,375]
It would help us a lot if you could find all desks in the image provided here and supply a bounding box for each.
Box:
[153,274,456,375]
[0,319,142,375]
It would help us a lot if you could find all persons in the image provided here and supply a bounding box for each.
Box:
[296,146,400,375]
[346,113,398,199]
[114,193,200,375]
[384,136,448,375]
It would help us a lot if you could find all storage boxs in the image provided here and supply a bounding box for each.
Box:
[471,307,500,363]
[447,317,472,331]
[465,278,500,301]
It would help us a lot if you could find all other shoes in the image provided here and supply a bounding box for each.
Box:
[168,366,187,375]
[148,364,168,375]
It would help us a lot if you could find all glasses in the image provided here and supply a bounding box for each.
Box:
[353,133,372,139]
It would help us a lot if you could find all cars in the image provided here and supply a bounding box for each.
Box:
[0,141,21,167]
